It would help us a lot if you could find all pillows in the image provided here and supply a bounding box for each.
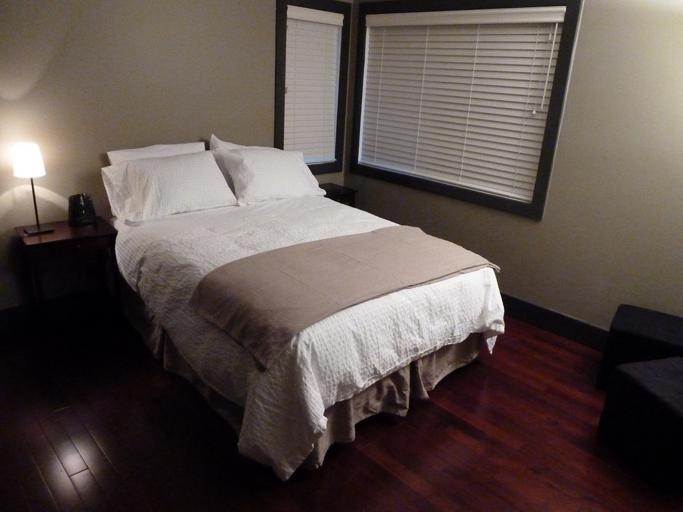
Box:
[98,132,325,230]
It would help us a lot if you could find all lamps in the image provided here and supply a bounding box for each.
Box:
[8,140,55,236]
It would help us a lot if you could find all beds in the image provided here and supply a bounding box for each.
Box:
[101,141,504,486]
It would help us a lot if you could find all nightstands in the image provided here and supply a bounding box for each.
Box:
[13,216,119,329]
[318,181,360,205]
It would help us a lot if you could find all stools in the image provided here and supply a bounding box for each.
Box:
[597,303,682,479]
